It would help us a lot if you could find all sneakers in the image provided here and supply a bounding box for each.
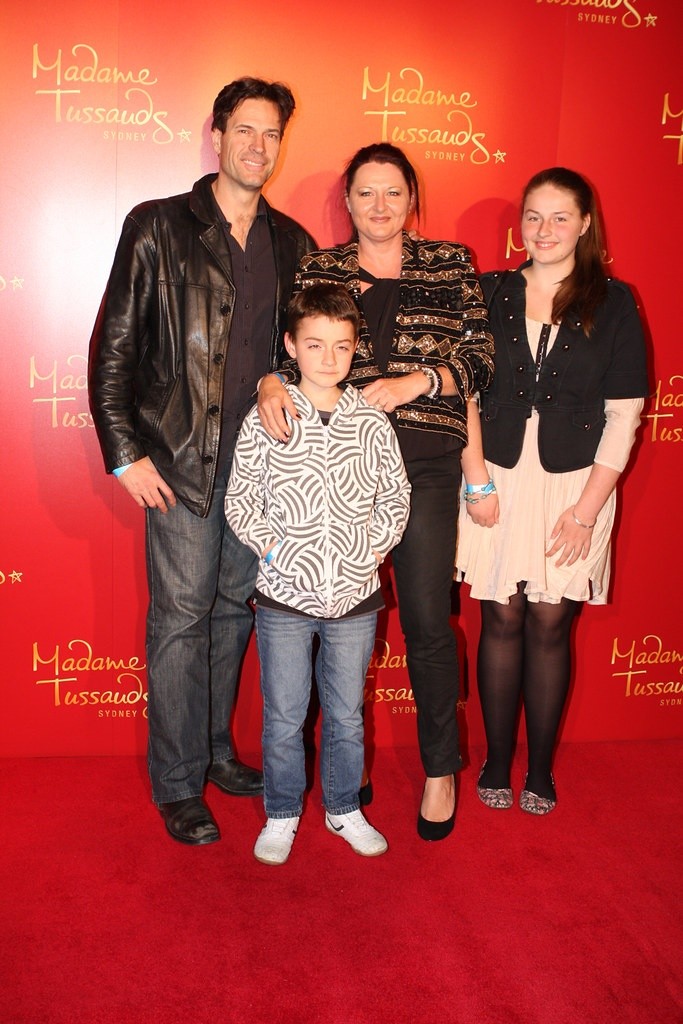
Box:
[254,813,300,865]
[325,810,389,858]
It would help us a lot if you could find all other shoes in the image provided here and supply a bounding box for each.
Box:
[475,757,514,809]
[416,772,458,842]
[362,776,377,805]
[519,770,558,816]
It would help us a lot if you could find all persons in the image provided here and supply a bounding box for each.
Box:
[256,143,494,841]
[225,285,411,865]
[455,168,648,814]
[88,79,319,846]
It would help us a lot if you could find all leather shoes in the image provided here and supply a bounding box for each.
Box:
[155,793,220,844]
[207,754,268,796]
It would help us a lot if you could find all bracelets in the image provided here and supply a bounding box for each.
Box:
[265,541,282,565]
[421,367,443,400]
[112,463,132,477]
[571,510,597,529]
[257,372,285,393]
[463,478,496,504]
